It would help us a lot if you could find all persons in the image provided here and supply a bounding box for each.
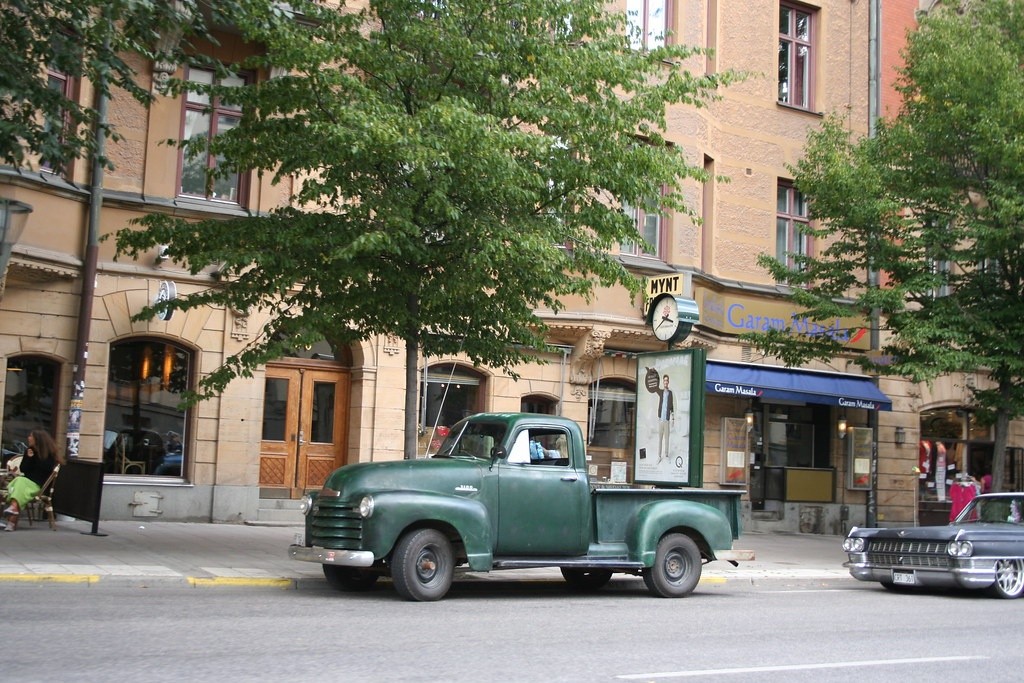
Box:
[931,441,948,501]
[919,439,933,500]
[542,434,568,459]
[981,463,992,494]
[0,429,67,531]
[107,431,183,474]
[645,366,675,464]
[1007,498,1024,523]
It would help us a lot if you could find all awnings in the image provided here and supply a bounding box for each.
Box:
[704,357,893,411]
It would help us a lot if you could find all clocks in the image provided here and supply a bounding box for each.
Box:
[646,293,699,343]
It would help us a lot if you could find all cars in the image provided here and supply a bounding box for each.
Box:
[842,492,1024,600]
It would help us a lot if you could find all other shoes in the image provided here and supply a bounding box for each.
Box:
[4,521,16,532]
[3,504,19,515]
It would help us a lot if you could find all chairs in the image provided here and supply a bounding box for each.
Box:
[6,454,61,531]
[115,434,145,475]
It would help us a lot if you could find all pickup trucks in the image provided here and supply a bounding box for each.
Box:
[288,411,749,604]
